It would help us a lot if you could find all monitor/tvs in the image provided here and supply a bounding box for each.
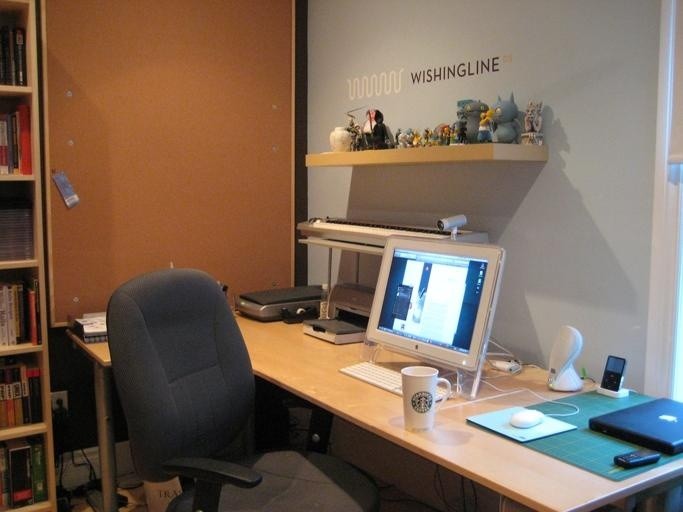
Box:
[365,234,508,399]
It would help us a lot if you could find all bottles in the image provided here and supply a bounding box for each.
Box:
[329,127,351,153]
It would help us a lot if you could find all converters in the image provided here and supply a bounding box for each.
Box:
[495,361,518,372]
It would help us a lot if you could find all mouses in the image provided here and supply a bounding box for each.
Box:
[511,410,544,428]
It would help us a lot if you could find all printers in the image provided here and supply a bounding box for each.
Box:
[302,282,376,345]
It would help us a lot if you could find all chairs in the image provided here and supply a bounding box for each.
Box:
[104,269,385,512]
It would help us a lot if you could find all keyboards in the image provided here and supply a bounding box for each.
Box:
[339,362,452,401]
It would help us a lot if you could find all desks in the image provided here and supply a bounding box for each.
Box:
[64,293,682,512]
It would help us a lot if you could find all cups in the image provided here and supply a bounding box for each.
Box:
[401,366,452,431]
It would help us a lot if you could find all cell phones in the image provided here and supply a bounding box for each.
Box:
[614,449,660,469]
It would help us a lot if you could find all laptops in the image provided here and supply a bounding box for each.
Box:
[590,397,683,456]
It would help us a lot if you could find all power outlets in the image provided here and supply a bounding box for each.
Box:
[48,391,68,415]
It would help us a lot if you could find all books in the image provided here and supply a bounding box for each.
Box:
[82,334,107,344]
[73,315,108,337]
[0,19,46,511]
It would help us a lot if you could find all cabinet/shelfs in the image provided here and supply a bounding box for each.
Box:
[1,0,63,512]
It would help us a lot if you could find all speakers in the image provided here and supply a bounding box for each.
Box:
[547,325,583,392]
